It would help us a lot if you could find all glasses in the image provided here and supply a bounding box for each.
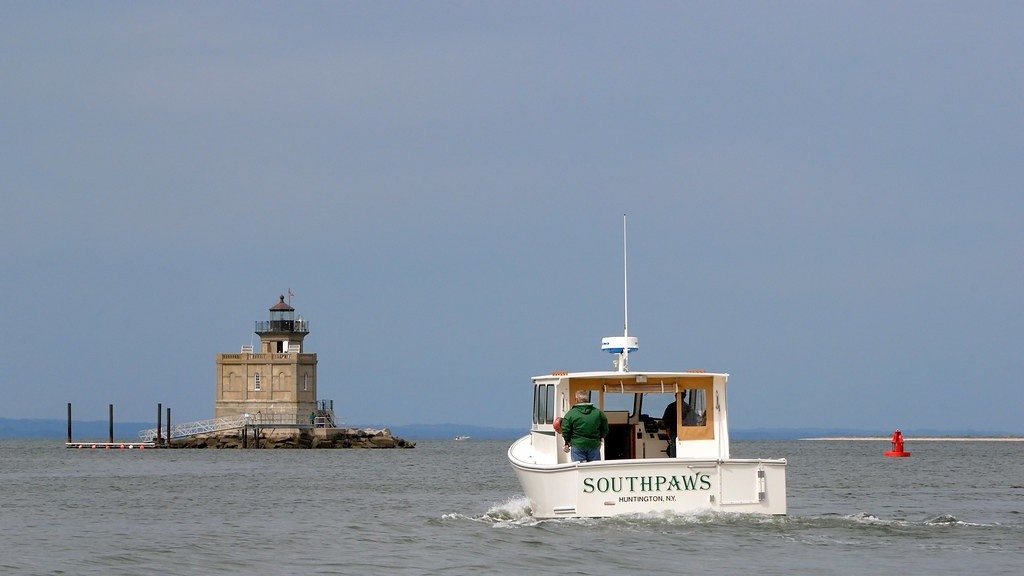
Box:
[575,397,578,399]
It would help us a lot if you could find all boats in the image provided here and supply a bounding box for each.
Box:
[455,435,471,441]
[507,215,788,522]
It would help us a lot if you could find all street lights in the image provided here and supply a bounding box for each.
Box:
[251,413,255,423]
[244,412,249,448]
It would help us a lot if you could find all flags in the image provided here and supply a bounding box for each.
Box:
[288,291,294,297]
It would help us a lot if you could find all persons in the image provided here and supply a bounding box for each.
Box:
[553,388,608,462]
[661,389,698,458]
[309,412,316,424]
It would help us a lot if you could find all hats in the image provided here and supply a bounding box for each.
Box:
[675,389,686,396]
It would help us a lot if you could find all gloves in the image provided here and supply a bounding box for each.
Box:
[666,428,672,439]
